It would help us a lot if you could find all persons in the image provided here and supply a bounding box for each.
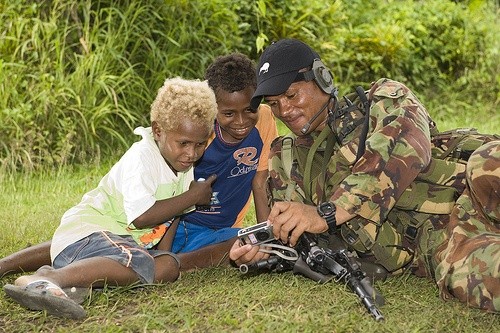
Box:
[0,53,280,283]
[5,77,220,320]
[227,40,500,317]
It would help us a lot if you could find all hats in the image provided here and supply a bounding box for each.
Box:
[250,38,321,113]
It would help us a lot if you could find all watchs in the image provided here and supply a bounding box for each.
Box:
[317,200,338,234]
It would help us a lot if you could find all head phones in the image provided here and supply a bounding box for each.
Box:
[302,58,336,94]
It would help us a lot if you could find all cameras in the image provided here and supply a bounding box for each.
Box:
[238,221,276,247]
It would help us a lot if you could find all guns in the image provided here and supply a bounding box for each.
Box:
[252,199,385,323]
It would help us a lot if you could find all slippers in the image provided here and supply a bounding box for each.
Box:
[5,284,86,319]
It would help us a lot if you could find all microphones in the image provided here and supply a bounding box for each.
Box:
[301,94,333,134]
